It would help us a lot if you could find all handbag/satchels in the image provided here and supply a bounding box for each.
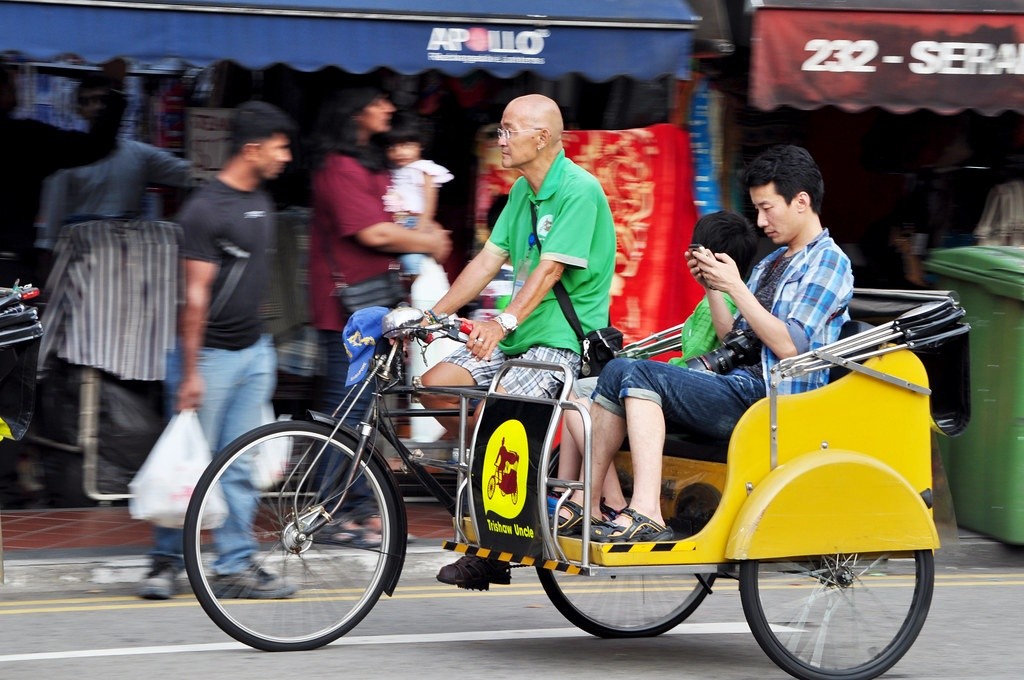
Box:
[128,406,230,531]
[580,327,623,377]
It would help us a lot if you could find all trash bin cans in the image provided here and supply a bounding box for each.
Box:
[925,245,1024,544]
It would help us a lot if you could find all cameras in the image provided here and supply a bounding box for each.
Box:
[684,328,762,376]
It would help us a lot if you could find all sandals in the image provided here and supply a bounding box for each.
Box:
[590,507,674,543]
[549,500,601,535]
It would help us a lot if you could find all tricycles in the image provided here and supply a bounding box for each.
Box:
[176,285,974,680]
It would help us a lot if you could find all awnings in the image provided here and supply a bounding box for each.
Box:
[743,0,1023,122]
[1,0,700,92]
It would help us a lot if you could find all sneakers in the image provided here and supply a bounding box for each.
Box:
[436,552,511,584]
[139,559,174,600]
[215,564,297,599]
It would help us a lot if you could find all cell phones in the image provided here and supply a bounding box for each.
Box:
[689,244,708,265]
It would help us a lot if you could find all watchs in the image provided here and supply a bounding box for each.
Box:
[492,313,518,335]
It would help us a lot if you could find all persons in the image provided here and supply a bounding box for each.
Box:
[416,88,622,591]
[973,181,1024,247]
[309,86,454,555]
[133,99,300,599]
[0,57,197,256]
[547,146,856,543]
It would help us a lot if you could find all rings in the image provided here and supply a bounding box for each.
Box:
[478,338,485,343]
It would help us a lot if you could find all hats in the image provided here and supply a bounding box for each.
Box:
[343,305,389,388]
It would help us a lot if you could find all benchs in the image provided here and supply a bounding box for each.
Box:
[617,316,878,468]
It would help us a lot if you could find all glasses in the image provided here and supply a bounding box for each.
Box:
[497,129,552,140]
